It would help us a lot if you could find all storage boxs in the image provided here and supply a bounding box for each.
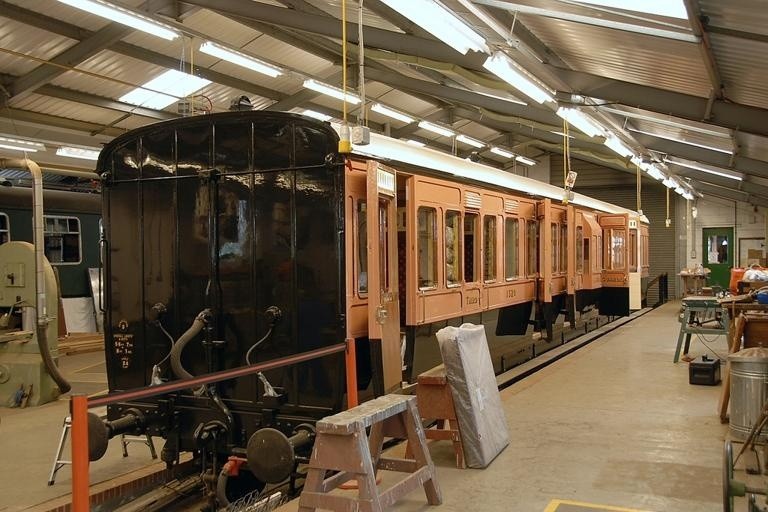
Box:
[687,355,723,386]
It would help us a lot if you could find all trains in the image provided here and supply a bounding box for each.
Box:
[0,181,103,297]
[87,95,649,512]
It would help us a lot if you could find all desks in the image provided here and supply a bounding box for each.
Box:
[673,266,768,364]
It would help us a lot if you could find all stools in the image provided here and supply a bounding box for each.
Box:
[296,364,469,512]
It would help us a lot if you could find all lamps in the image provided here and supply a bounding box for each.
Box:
[300,79,360,104]
[55,145,101,160]
[58,0,182,41]
[455,133,540,168]
[370,104,416,124]
[197,42,283,78]
[381,0,490,55]
[602,133,697,202]
[481,51,556,104]
[554,104,606,138]
[418,120,457,137]
